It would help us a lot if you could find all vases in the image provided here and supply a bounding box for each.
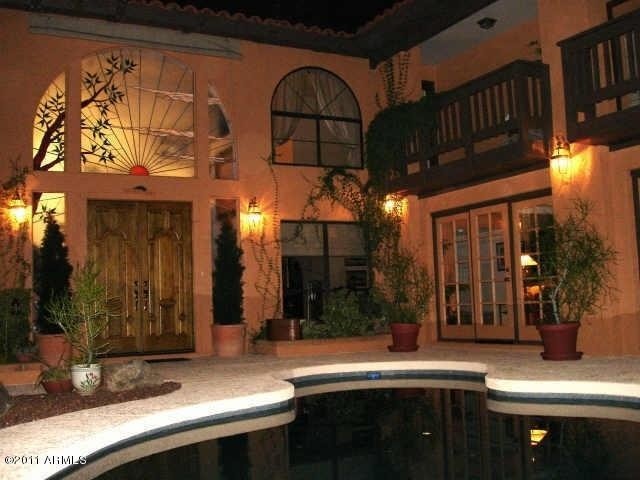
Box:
[268,316,300,339]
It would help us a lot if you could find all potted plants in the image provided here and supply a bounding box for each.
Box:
[358,207,438,352]
[211,213,250,361]
[28,210,117,394]
[526,195,616,362]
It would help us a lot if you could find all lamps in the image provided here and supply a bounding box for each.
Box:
[551,133,572,175]
